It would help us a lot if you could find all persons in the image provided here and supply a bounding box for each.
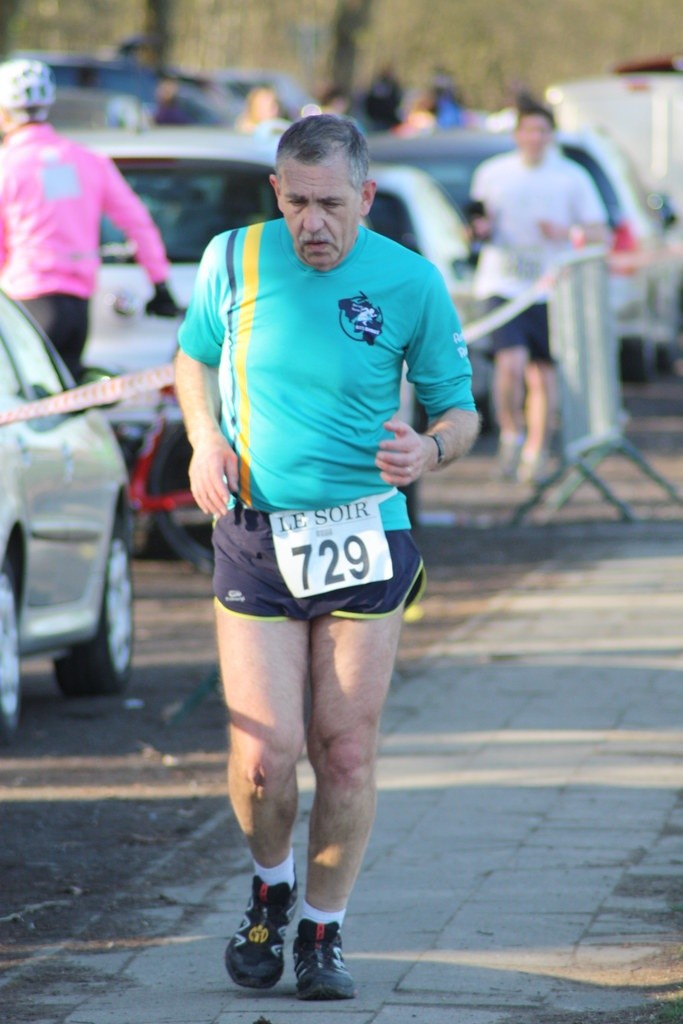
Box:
[469,90,618,485]
[178,115,483,999]
[431,66,463,129]
[320,86,350,115]
[365,56,403,129]
[92,35,159,102]
[149,77,196,124]
[233,83,282,135]
[0,56,183,382]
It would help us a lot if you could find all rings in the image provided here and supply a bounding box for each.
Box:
[406,467,413,472]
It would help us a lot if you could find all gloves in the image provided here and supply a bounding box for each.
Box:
[146,285,176,317]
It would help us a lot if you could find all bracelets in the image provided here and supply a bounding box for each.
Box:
[427,432,446,463]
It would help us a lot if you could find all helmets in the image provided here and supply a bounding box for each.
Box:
[0,59,56,108]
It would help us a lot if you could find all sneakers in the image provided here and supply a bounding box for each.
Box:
[292,918,354,999]
[225,863,299,990]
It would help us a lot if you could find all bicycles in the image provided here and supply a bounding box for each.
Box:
[35,301,230,579]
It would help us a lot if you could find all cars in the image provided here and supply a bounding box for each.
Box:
[20,50,681,555]
[3,295,140,743]
